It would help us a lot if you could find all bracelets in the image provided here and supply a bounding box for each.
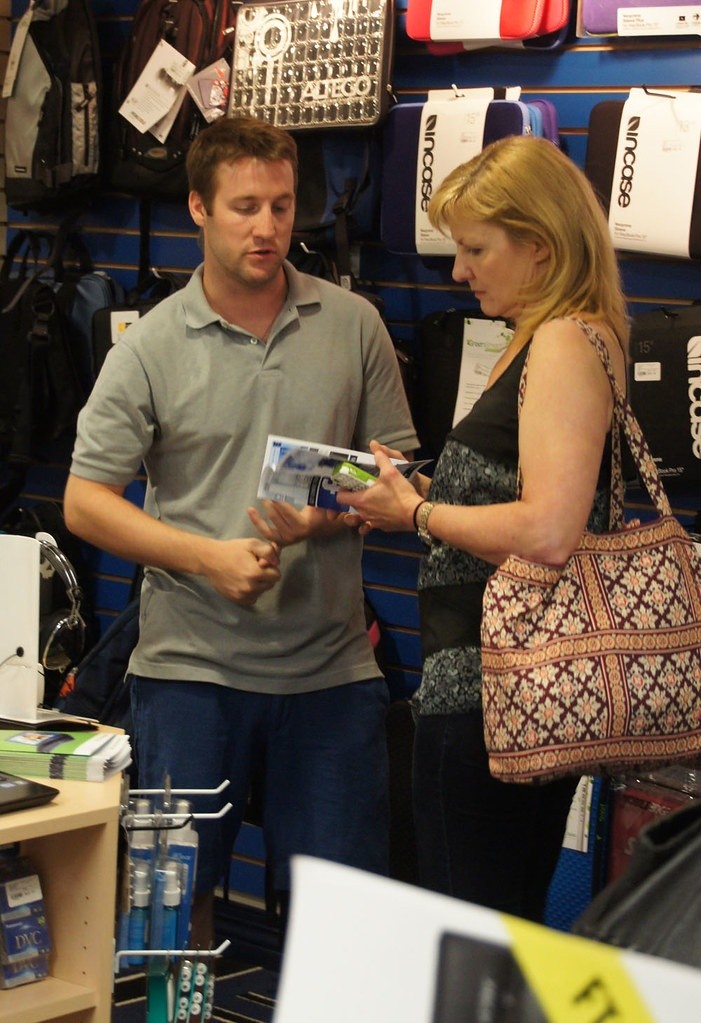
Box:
[413,499,430,530]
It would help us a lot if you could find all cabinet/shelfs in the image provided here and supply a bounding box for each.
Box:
[2,706,126,1023]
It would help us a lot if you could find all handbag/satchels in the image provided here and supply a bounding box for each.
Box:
[482,317,700,783]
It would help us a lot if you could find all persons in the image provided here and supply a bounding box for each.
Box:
[61,114,426,1023]
[335,132,639,931]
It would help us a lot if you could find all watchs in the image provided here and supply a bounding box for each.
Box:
[416,501,441,548]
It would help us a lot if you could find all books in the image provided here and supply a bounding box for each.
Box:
[0,728,134,783]
